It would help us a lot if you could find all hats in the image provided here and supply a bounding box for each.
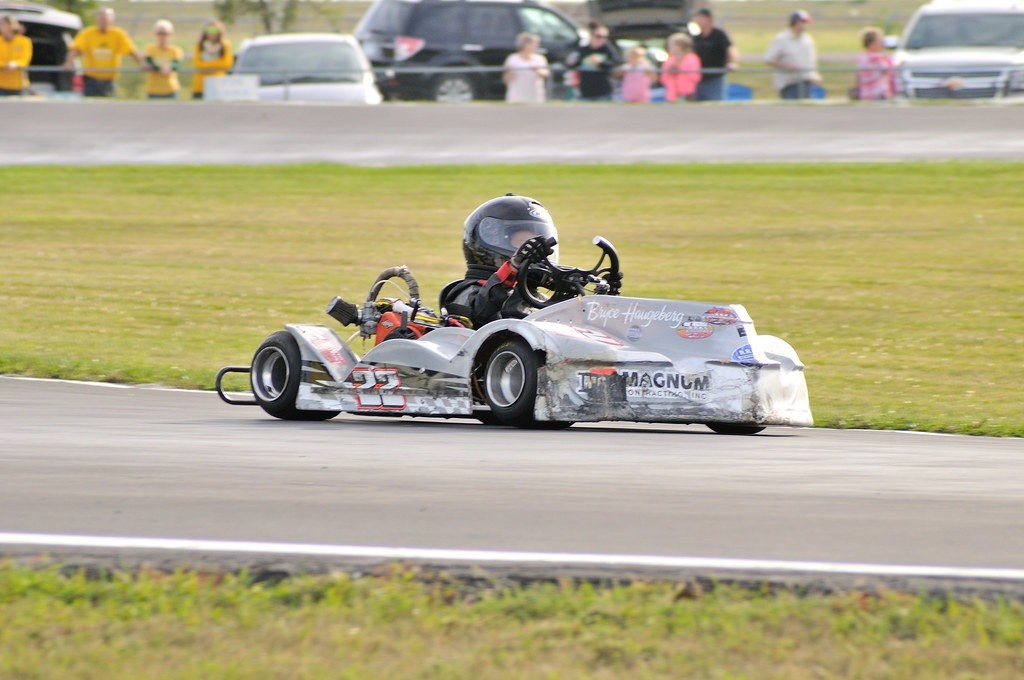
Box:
[152,19,173,35]
[788,10,815,26]
[691,8,711,17]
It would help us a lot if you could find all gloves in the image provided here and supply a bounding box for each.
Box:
[510,235,554,270]
[602,272,624,288]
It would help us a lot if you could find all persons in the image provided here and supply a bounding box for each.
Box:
[144,20,184,99]
[855,27,897,100]
[502,33,552,103]
[659,8,739,103]
[576,20,620,100]
[62,7,149,97]
[614,47,658,102]
[766,10,821,98]
[0,14,33,96]
[192,21,233,98]
[439,193,623,331]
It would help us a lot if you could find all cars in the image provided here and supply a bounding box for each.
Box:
[204,33,394,106]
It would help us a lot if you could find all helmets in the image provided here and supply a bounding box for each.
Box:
[462,192,559,270]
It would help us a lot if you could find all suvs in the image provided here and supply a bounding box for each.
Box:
[353,0,618,102]
[0,4,83,96]
[883,0,1024,99]
[588,0,702,59]
